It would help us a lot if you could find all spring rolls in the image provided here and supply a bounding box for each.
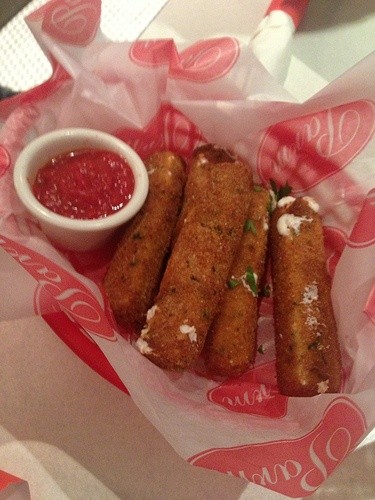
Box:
[208,184,274,378]
[134,161,254,371]
[105,149,185,330]
[270,194,341,398]
[178,150,230,239]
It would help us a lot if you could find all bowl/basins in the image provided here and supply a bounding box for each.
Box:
[13,127,148,249]
